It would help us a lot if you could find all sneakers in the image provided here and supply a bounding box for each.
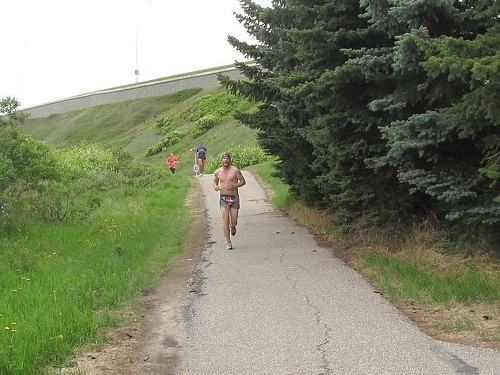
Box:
[226,241,232,250]
[231,227,236,236]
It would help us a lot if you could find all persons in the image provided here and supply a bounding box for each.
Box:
[167,153,178,174]
[212,153,246,250]
[196,142,207,177]
